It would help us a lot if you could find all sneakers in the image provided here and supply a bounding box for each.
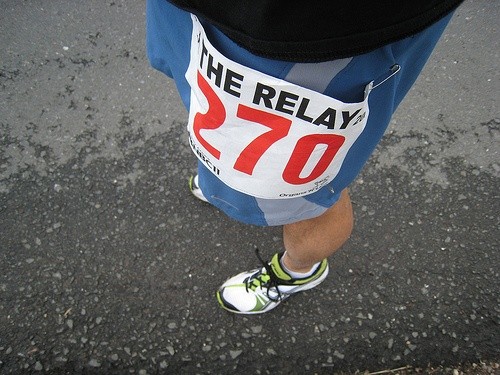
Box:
[188,175,212,205]
[216,246,329,314]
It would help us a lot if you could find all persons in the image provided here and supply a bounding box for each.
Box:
[146,1,464,314]
